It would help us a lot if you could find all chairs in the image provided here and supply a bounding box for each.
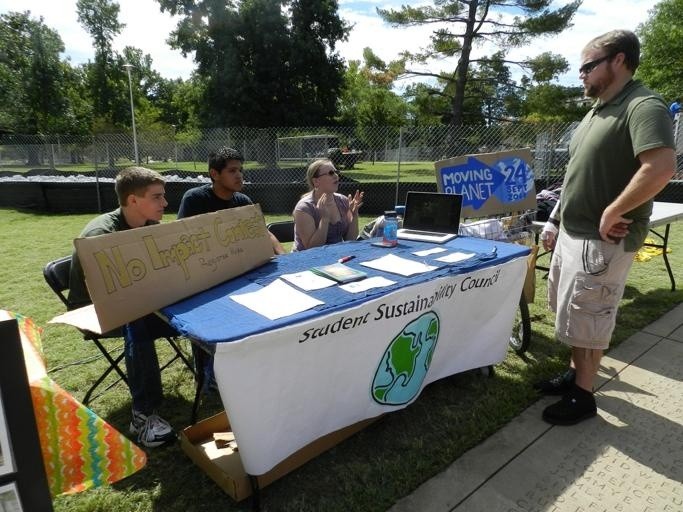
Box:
[265,219,295,243]
[41,254,198,408]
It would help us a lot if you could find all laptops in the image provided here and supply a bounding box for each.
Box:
[397,191,464,243]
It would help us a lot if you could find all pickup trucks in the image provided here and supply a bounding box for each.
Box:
[308,147,363,169]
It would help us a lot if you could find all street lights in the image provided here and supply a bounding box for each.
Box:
[122,61,140,166]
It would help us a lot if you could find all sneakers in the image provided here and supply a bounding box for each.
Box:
[128,409,173,449]
[532,371,585,398]
[540,384,597,426]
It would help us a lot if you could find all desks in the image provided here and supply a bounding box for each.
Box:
[151,235,534,512]
[0,310,148,512]
[534,201,683,293]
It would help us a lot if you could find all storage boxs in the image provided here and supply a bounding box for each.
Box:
[433,147,540,219]
[179,394,386,503]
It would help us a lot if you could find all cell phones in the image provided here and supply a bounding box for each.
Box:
[371,242,395,248]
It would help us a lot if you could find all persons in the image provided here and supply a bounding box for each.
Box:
[176,145,288,257]
[533,29,677,427]
[668,98,683,119]
[291,159,367,252]
[65,165,220,450]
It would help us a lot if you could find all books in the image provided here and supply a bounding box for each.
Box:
[308,263,367,284]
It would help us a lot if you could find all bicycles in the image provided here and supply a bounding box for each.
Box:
[455,207,538,355]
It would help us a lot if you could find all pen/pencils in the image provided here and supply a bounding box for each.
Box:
[338,256,356,263]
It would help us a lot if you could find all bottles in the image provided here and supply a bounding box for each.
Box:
[381,211,397,246]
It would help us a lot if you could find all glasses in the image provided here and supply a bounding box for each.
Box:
[313,169,340,178]
[579,53,613,73]
